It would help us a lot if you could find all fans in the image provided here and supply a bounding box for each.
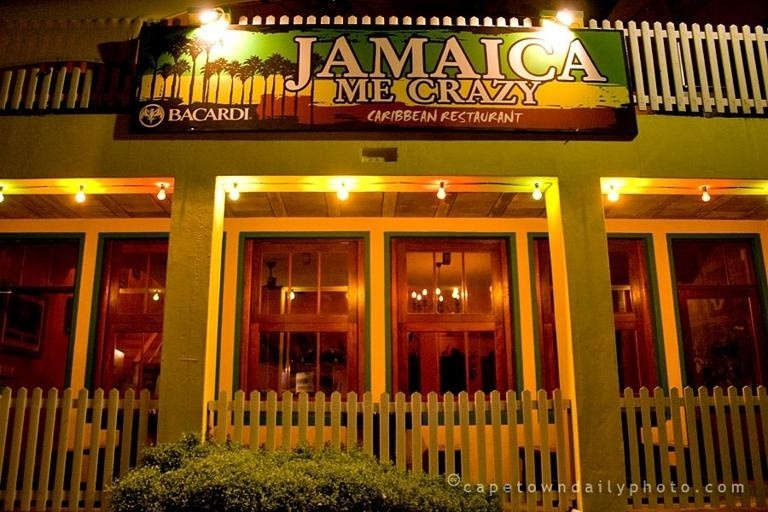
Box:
[262,261,285,290]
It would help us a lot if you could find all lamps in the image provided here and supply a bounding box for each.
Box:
[152,290,159,300]
[228,182,240,202]
[401,260,464,317]
[286,288,296,300]
[74,184,87,204]
[336,182,350,201]
[607,185,620,202]
[701,186,711,203]
[436,180,447,201]
[156,183,167,201]
[532,182,543,201]
